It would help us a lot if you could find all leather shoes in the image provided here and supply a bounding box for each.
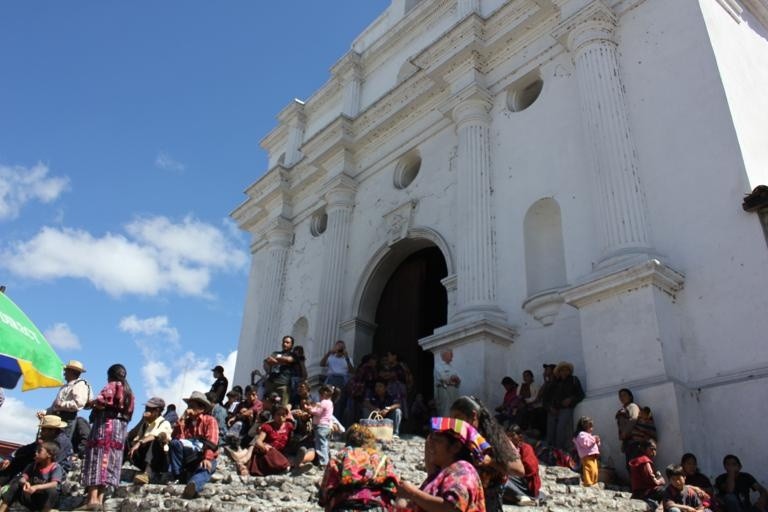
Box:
[182,481,196,499]
[74,502,99,512]
[164,477,181,485]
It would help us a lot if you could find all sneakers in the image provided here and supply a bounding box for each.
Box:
[134,472,153,484]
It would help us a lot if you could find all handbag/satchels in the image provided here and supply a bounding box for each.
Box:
[249,444,290,475]
[360,411,394,442]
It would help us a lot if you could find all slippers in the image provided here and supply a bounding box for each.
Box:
[288,463,313,476]
[223,446,239,463]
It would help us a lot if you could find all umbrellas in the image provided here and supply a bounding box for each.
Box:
[0,283,68,400]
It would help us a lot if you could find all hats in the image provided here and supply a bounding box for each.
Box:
[554,361,574,377]
[39,414,68,430]
[63,359,87,373]
[142,397,165,409]
[182,390,211,412]
[211,365,224,372]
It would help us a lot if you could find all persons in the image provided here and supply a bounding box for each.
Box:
[316,344,586,510]
[571,416,603,490]
[615,388,658,489]
[628,436,666,512]
[680,453,716,496]
[1,335,357,510]
[664,462,711,512]
[642,405,653,425]
[707,454,767,510]
